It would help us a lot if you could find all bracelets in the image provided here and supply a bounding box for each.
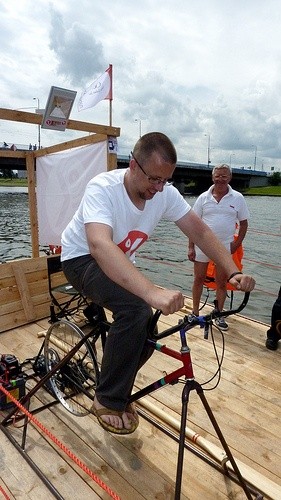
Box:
[227,272,243,282]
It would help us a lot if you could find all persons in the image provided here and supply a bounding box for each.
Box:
[188,164,250,330]
[266,286,281,351]
[60,132,256,435]
[29,143,32,150]
[33,144,37,150]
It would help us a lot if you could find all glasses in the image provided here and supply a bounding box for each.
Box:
[130,150,175,186]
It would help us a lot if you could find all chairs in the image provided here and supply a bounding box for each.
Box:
[198,223,244,318]
[47,255,93,330]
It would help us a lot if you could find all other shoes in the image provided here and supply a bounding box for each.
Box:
[265,338,277,351]
[215,317,229,330]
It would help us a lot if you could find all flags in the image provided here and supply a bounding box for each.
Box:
[78,66,112,111]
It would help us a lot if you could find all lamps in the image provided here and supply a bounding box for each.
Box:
[35,85,77,131]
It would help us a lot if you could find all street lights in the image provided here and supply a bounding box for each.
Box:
[204,134,211,167]
[33,97,41,150]
[135,118,141,139]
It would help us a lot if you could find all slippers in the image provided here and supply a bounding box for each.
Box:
[91,402,139,435]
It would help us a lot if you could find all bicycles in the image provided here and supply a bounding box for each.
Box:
[0,255,254,500]
